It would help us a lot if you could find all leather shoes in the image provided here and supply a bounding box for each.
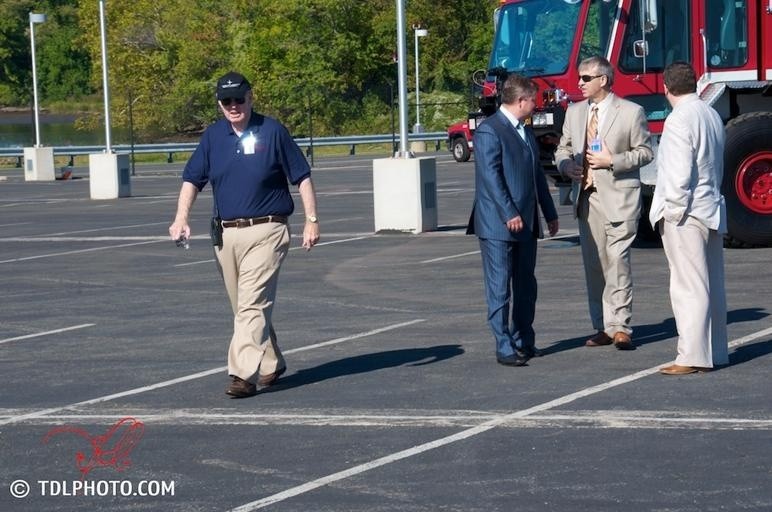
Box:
[518,345,544,357]
[613,332,633,350]
[257,366,286,386]
[660,365,699,375]
[497,353,529,366]
[586,331,613,346]
[225,375,256,397]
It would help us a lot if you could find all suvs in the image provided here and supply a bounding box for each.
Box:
[448,113,482,160]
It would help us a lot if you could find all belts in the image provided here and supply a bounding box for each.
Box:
[223,216,287,228]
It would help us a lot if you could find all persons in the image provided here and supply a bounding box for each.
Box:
[466,74,559,367]
[648,59,730,376]
[554,55,654,349]
[168,71,320,398]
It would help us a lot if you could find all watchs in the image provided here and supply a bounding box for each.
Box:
[304,216,320,223]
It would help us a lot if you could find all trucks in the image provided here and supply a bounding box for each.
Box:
[479,0,771,245]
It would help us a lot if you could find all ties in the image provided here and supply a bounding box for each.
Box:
[518,121,529,147]
[582,106,598,191]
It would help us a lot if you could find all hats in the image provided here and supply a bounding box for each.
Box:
[217,71,251,100]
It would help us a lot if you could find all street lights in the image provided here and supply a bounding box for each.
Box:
[409,23,428,153]
[22,11,57,183]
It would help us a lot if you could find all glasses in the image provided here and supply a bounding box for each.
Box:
[220,97,245,106]
[578,75,603,82]
